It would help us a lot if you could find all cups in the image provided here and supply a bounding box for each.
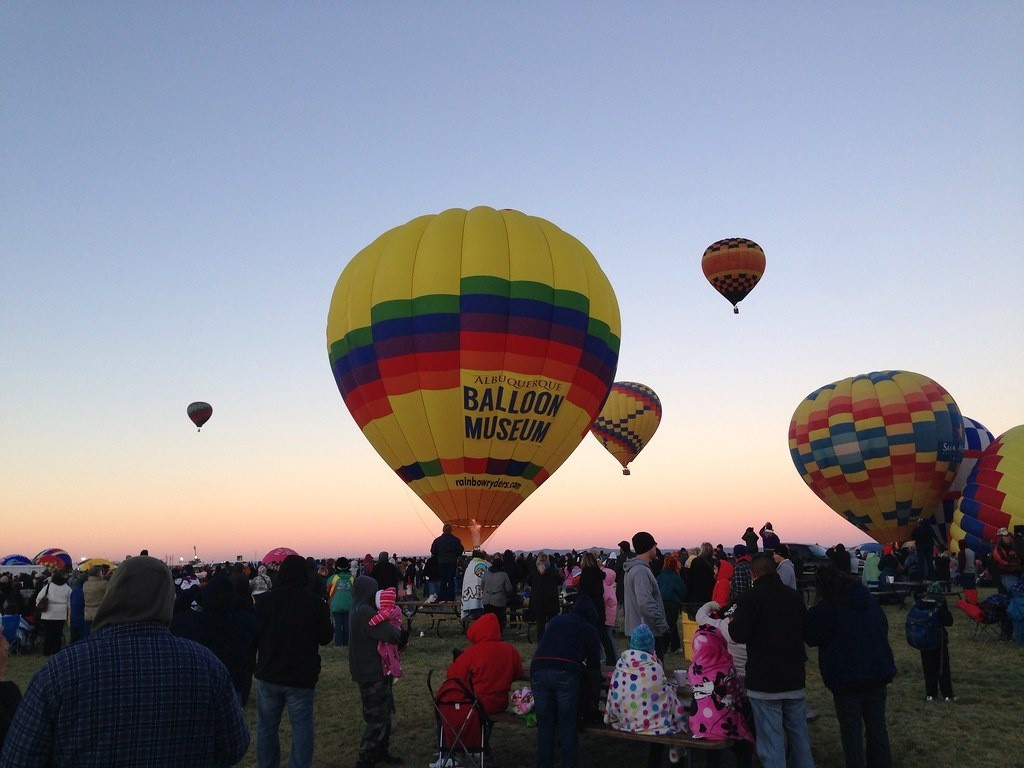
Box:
[674,670,687,686]
[420,632,424,637]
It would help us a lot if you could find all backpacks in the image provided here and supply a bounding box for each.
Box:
[905,603,939,650]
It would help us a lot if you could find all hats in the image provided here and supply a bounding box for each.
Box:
[630,624,655,655]
[617,541,630,551]
[774,544,791,560]
[335,556,351,573]
[997,527,1009,536]
[632,531,656,554]
[765,522,773,531]
[927,581,947,595]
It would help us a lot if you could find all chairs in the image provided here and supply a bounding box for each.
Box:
[956,589,1001,643]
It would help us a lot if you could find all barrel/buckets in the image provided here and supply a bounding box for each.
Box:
[886,576,894,583]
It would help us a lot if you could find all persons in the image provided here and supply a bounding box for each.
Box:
[804,563,898,768]
[0,624,22,752]
[728,552,815,768]
[913,581,960,702]
[0,555,250,768]
[0,517,1024,768]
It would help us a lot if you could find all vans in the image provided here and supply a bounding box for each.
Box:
[780,541,828,584]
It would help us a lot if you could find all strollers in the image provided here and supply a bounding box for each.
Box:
[427,664,499,768]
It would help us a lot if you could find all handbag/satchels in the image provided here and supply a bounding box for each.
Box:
[37,596,49,612]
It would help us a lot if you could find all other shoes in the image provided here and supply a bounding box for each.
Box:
[926,696,935,702]
[946,695,960,703]
[428,756,459,768]
[380,757,404,768]
[465,752,481,758]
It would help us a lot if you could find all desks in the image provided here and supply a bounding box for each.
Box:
[885,579,950,610]
[600,666,694,768]
[395,601,461,632]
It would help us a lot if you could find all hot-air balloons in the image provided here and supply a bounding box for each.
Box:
[321,203,623,557]
[262,547,299,563]
[786,369,967,550]
[31,548,74,577]
[186,401,214,433]
[945,424,1024,565]
[588,381,664,475]
[946,416,998,510]
[700,236,767,315]
[72,558,115,573]
[0,553,32,566]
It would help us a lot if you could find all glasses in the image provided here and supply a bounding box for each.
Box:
[655,543,658,546]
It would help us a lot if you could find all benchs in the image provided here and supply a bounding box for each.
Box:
[917,591,964,599]
[557,720,735,768]
[402,609,460,638]
[870,590,915,611]
[504,610,536,644]
